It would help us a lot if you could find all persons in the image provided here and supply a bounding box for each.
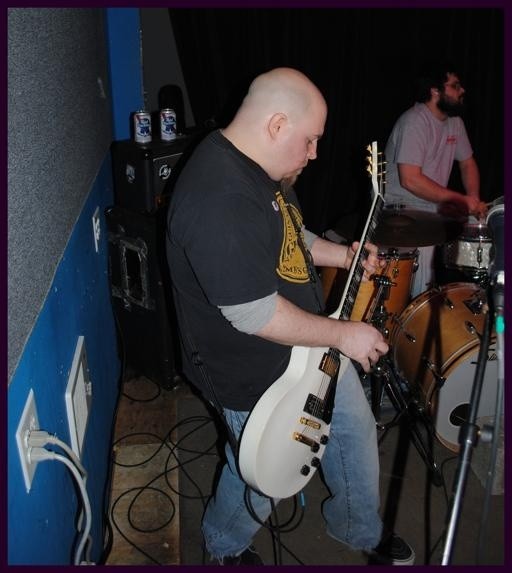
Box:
[379,66,488,301]
[162,64,417,567]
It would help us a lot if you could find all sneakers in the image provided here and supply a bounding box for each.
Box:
[209,533,263,568]
[330,520,417,566]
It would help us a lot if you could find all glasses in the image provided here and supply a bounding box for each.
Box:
[440,82,461,90]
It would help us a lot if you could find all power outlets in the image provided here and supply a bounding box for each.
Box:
[17,386,43,494]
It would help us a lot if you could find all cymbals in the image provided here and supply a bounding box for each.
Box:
[333,210,458,247]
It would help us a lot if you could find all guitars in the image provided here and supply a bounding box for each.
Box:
[235,142,388,497]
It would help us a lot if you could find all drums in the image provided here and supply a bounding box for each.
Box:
[391,282,505,454]
[321,228,421,343]
[443,224,494,271]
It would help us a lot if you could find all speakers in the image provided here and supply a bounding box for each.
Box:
[110,128,202,216]
[105,205,181,392]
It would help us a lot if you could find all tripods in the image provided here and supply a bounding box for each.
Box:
[438,269,505,566]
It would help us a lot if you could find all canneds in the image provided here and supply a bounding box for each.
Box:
[133,111,153,144]
[160,108,177,141]
